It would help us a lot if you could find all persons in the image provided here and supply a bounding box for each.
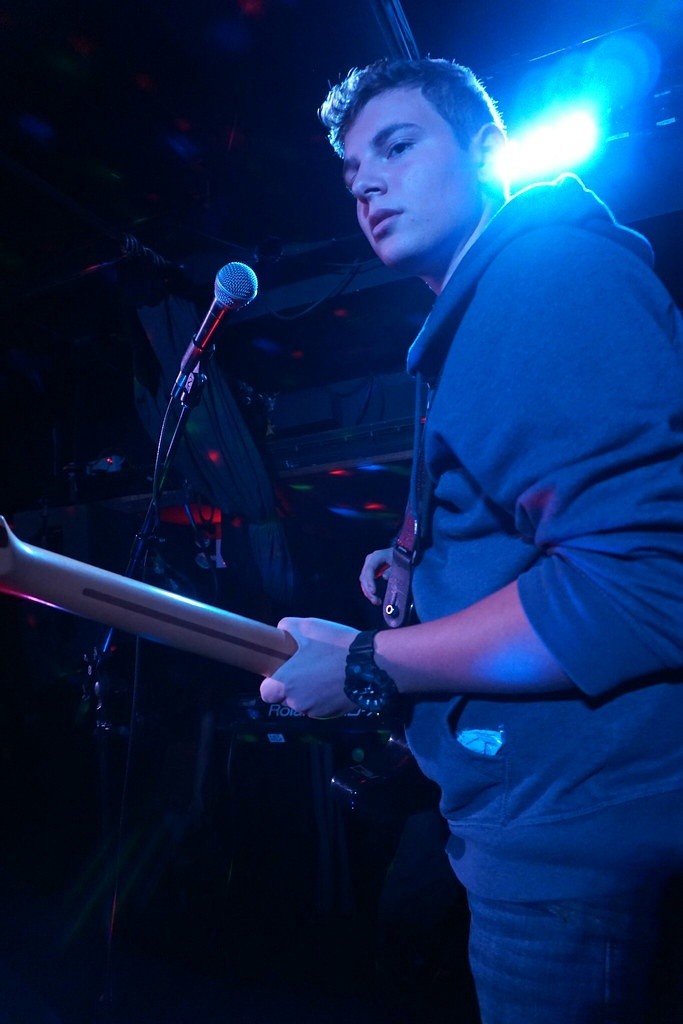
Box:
[260,52,683,1024]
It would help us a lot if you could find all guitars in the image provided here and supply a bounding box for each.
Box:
[1,510,419,761]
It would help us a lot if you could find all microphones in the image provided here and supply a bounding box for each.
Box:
[169,262,258,398]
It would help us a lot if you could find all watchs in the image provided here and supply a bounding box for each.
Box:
[343,630,398,713]
